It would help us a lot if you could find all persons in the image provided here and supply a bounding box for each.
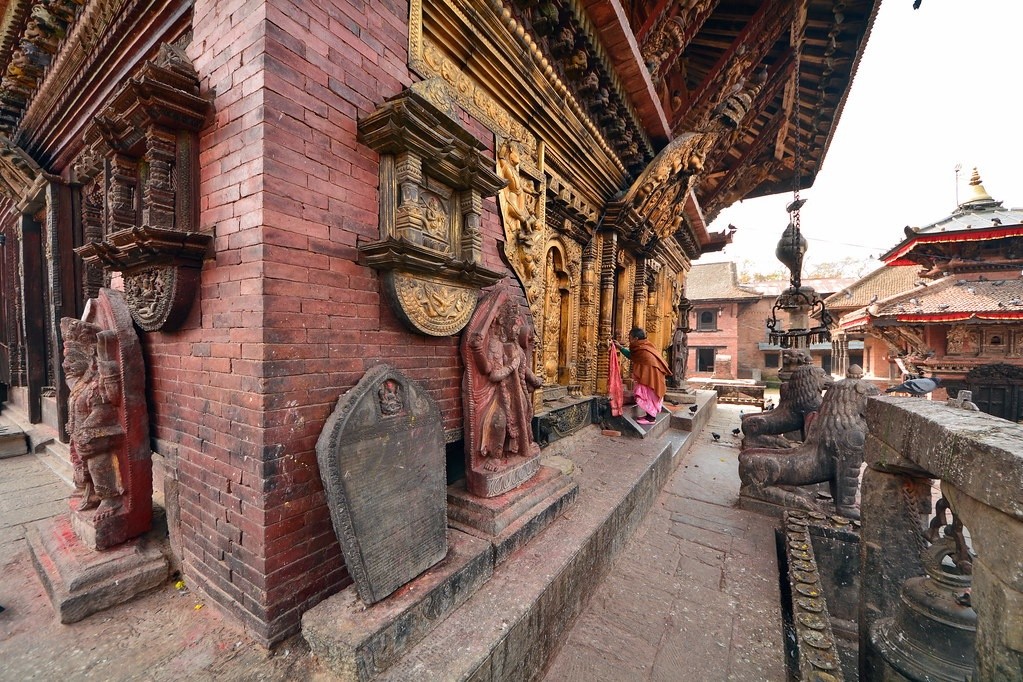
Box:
[482,299,540,472]
[612,327,673,426]
[55,314,123,525]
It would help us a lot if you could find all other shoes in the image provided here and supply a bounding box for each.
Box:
[637,418,655,425]
[637,415,647,420]
